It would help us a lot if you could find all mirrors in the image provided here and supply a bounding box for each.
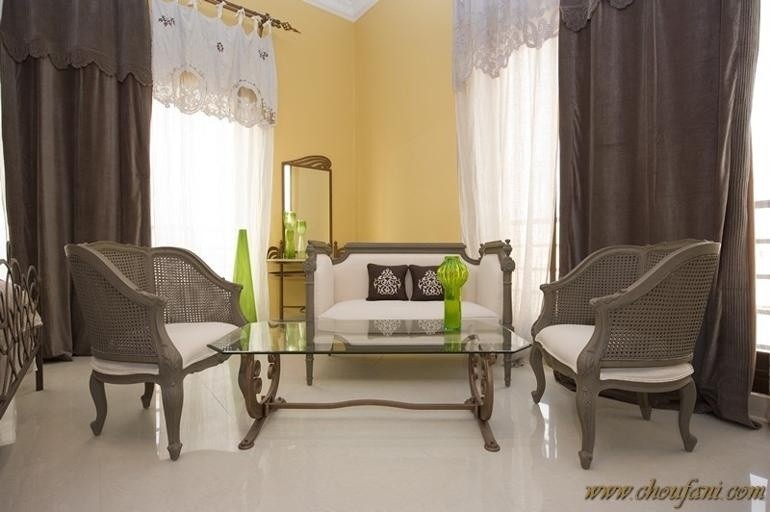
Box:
[281,155,333,256]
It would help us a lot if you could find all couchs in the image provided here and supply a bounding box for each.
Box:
[304,239,516,388]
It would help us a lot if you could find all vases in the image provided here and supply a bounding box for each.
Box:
[296,219,307,259]
[437,256,469,328]
[283,211,296,259]
[232,229,257,321]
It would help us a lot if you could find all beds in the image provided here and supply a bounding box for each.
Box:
[205,320,532,453]
[0,258,44,422]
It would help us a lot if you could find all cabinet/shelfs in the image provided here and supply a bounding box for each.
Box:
[266,240,332,321]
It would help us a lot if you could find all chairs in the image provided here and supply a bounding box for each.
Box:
[529,239,722,470]
[64,240,251,462]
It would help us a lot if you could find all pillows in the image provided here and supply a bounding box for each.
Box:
[366,263,408,301]
[408,264,444,301]
